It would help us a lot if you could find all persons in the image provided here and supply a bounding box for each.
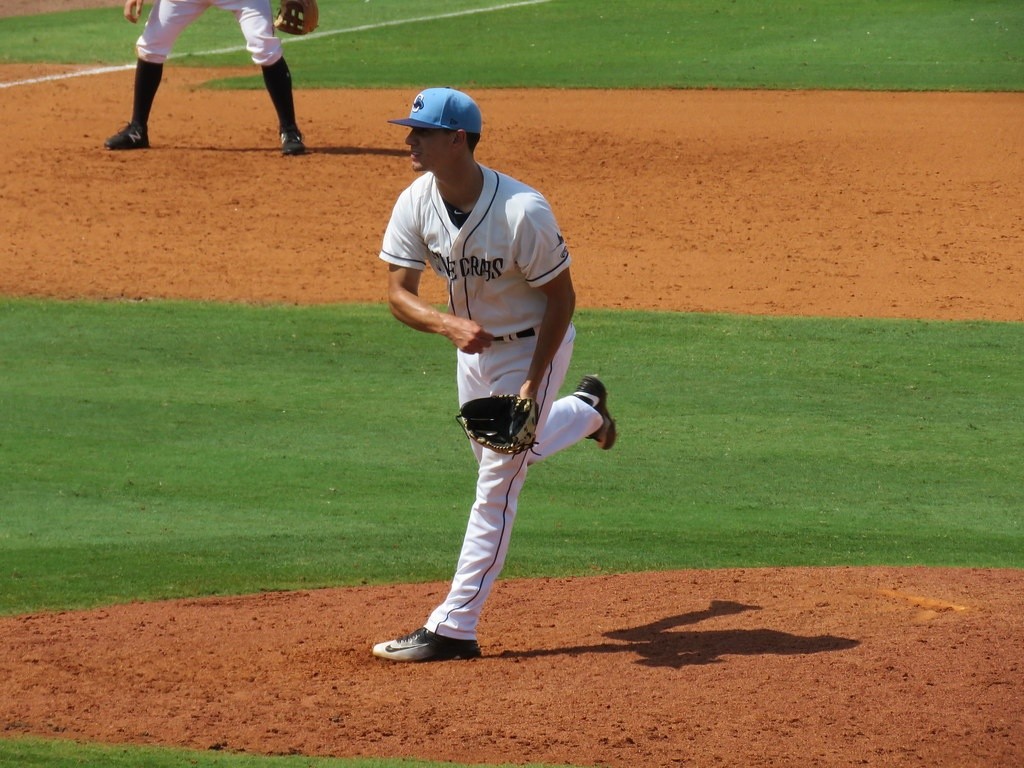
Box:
[104,0,306,155]
[373,88,615,661]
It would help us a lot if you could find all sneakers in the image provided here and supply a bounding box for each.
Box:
[280,130,304,154]
[572,374,616,450]
[104,121,150,150]
[373,626,481,660]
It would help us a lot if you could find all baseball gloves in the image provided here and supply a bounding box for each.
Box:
[456,394,542,457]
[274,1,320,35]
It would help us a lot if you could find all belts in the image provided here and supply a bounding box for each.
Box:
[493,328,535,342]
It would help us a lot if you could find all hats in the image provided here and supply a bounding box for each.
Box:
[386,85,482,133]
